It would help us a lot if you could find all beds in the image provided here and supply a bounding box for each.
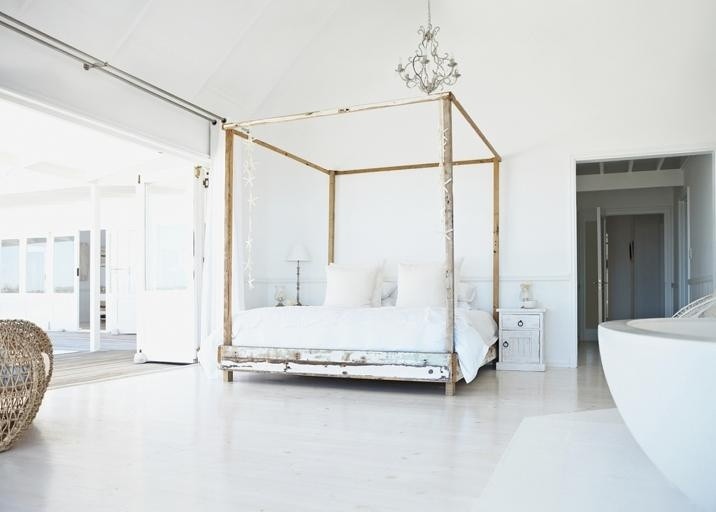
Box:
[217,277,498,396]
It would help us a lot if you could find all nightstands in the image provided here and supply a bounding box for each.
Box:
[495,308,546,371]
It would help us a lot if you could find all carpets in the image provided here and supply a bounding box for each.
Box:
[482,407,706,511]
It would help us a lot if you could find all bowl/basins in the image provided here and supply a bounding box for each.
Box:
[523,301,536,309]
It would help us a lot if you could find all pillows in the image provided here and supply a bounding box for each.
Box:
[322,262,476,309]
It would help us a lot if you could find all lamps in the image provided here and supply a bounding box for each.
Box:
[286,245,313,306]
[393,0,462,96]
